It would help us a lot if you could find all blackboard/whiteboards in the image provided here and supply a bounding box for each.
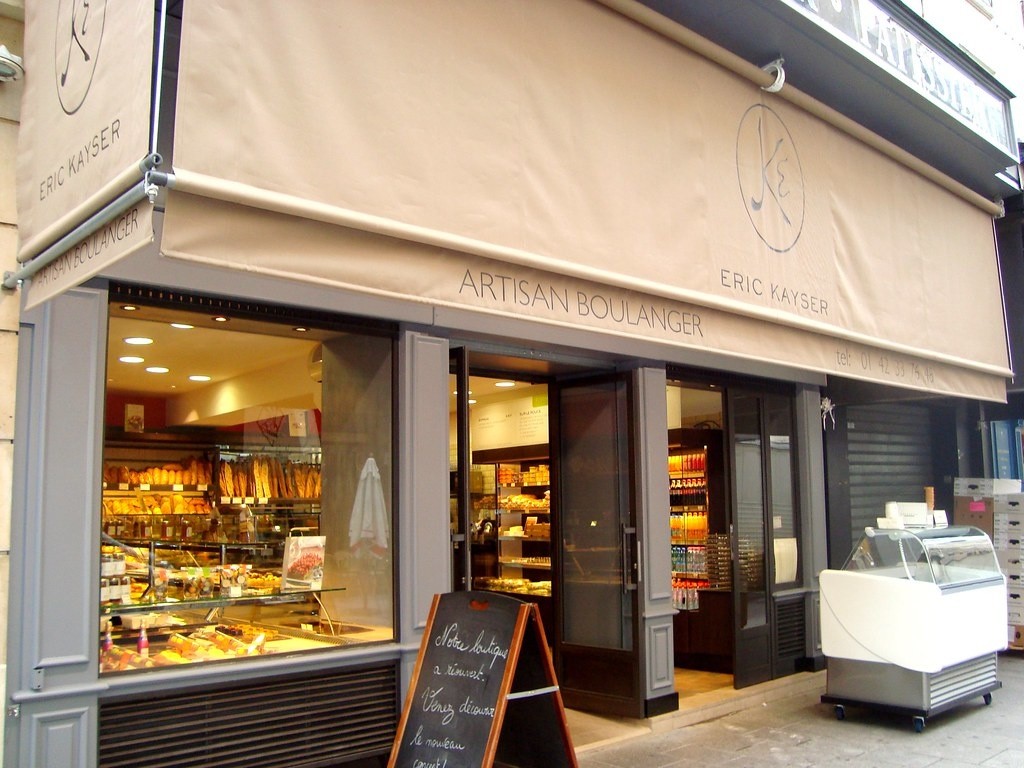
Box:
[385,590,529,768]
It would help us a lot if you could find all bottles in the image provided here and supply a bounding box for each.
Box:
[102,622,113,652]
[141,520,151,539]
[670,512,706,539]
[667,453,704,471]
[671,578,710,609]
[238,504,254,543]
[104,520,123,537]
[162,521,174,540]
[670,547,707,573]
[137,619,148,659]
[181,520,192,538]
[669,478,706,505]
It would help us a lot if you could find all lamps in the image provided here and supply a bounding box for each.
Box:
[0,44,25,82]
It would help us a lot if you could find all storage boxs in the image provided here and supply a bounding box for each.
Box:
[125,404,144,432]
[953,476,1024,647]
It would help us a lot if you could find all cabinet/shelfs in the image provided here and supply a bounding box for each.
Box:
[471,427,734,674]
